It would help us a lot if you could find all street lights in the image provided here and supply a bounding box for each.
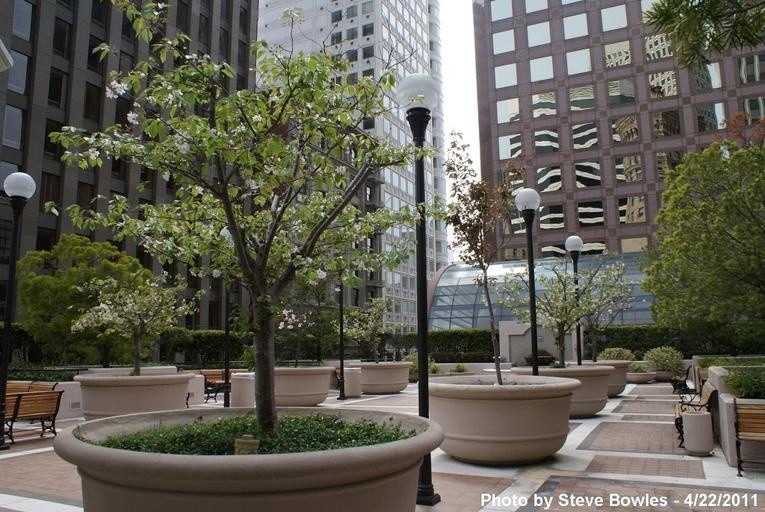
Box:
[515,187,541,376]
[219,225,231,407]
[335,252,348,400]
[565,235,584,366]
[0,165,37,450]
[395,71,439,506]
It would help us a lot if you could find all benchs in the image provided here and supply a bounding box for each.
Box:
[178,368,342,404]
[730,396,764,478]
[3,377,65,445]
[668,362,694,395]
[672,382,720,449]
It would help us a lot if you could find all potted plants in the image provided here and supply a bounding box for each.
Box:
[46,0,446,512]
[273,124,638,466]
